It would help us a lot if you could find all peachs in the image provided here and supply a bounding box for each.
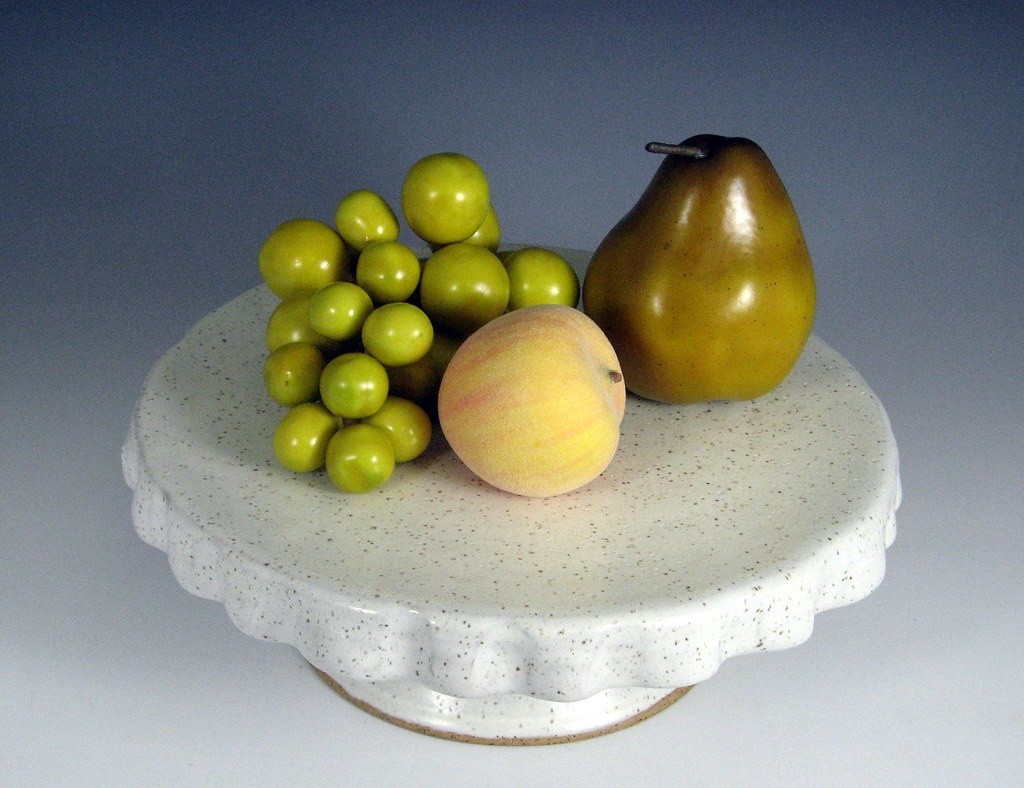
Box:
[437,307,626,499]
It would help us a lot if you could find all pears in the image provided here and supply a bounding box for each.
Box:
[579,134,816,405]
